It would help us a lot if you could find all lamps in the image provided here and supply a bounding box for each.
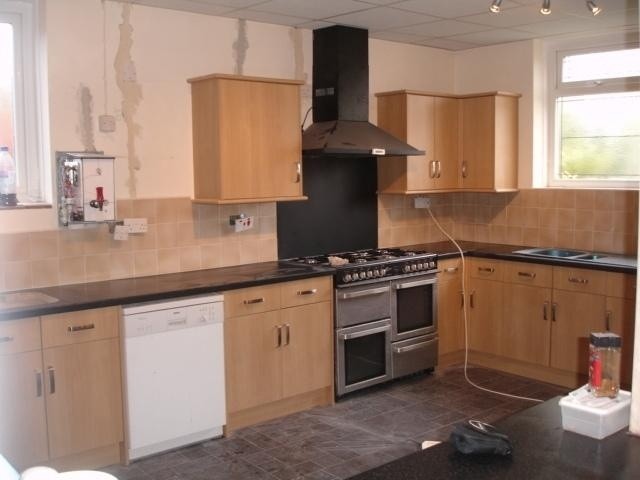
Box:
[489,0,603,17]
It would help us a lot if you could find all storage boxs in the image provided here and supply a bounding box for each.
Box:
[559,383,632,441]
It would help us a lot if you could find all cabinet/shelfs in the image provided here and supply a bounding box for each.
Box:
[221,275,335,438]
[437,257,636,389]
[0,305,129,480]
[457,91,521,193]
[374,89,458,195]
[187,74,308,206]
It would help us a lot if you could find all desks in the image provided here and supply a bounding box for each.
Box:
[343,395,640,480]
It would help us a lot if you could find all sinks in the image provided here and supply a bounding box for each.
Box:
[511,247,610,262]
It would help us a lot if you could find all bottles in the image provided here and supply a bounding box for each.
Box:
[589,332,622,398]
[1,145,21,209]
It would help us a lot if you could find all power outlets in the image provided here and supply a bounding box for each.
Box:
[235,216,253,232]
[414,197,431,209]
[114,218,148,241]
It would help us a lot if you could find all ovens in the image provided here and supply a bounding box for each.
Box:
[391,276,438,343]
[334,319,392,398]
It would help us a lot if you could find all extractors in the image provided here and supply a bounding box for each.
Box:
[294,24,427,159]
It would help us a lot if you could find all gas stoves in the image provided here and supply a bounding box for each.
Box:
[279,248,442,289]
